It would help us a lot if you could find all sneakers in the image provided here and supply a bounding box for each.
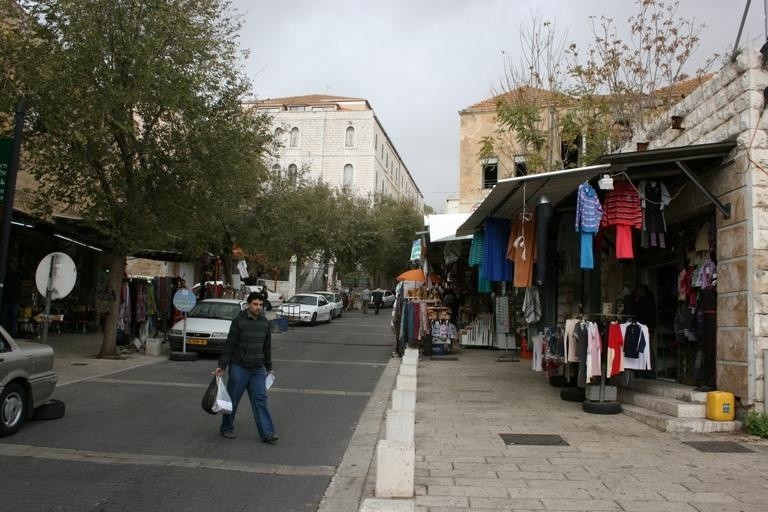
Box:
[265,432,279,443]
[221,430,235,438]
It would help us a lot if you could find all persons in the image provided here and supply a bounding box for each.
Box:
[359,286,371,314]
[259,285,268,301]
[169,277,214,324]
[371,288,383,315]
[634,283,657,379]
[214,292,281,444]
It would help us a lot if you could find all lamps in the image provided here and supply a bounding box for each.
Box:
[597,171,617,190]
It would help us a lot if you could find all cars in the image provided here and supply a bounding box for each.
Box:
[368,287,396,308]
[193,280,224,289]
[276,292,335,325]
[315,290,344,319]
[162,298,252,354]
[247,285,285,311]
[0,323,58,433]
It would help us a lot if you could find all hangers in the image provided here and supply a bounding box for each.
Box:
[403,299,453,326]
[582,176,593,189]
[575,316,636,328]
[518,206,533,223]
